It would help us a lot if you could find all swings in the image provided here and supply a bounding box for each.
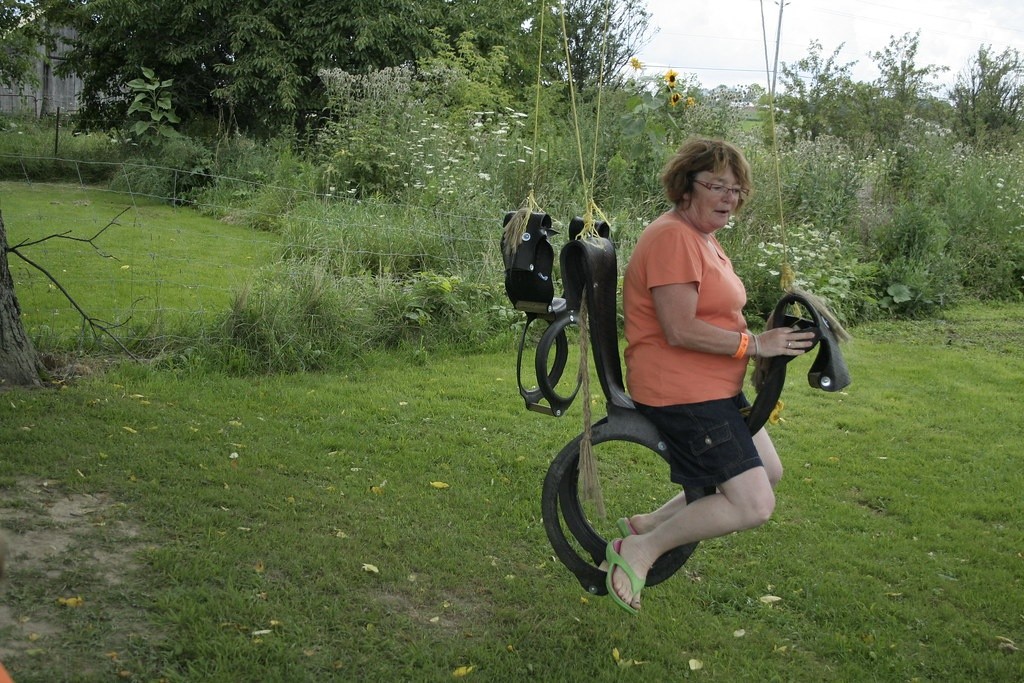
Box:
[542,0,852,597]
[498,1,610,417]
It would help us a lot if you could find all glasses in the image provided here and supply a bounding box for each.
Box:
[694,178,749,200]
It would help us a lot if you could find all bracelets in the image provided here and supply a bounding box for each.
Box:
[733,333,749,360]
[752,334,761,358]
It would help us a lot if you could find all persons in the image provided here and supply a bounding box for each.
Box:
[605,140,815,617]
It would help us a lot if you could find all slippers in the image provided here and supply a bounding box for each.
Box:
[616,517,638,537]
[606,537,646,616]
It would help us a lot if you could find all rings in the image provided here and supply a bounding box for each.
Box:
[787,341,791,348]
